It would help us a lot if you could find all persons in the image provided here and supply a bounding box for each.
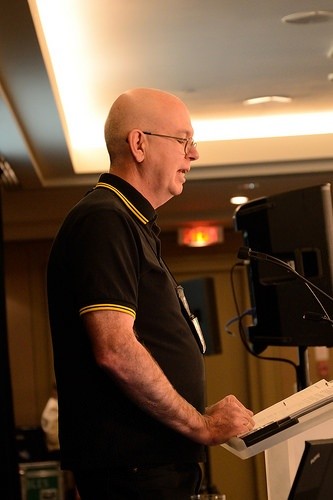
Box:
[41,383,65,463]
[44,87,253,500]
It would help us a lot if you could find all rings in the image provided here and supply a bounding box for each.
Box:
[246,420,250,428]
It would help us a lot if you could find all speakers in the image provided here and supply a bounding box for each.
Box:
[232,183,332,346]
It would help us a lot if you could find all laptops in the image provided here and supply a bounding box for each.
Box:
[287,439,333,500]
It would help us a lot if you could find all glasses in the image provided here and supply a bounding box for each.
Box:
[126,130,201,158]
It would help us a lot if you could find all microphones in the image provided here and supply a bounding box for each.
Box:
[237,246,333,301]
